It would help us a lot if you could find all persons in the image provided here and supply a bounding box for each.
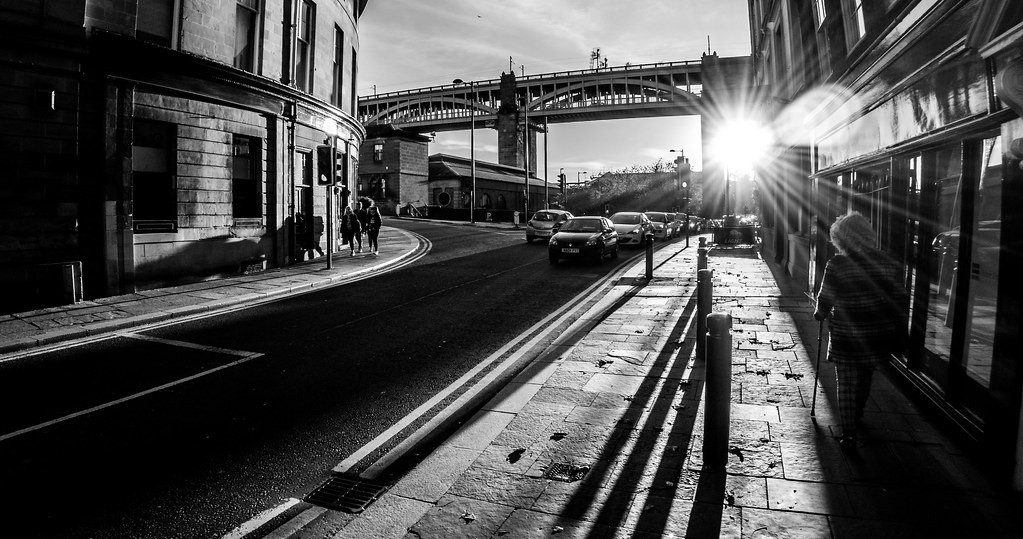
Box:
[812,211,899,450]
[340,202,382,256]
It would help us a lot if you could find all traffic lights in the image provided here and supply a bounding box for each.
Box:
[317,145,334,187]
[678,164,690,190]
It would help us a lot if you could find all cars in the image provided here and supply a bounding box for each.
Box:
[526,210,573,242]
[645,211,672,242]
[667,211,759,238]
[549,216,620,265]
[610,212,657,247]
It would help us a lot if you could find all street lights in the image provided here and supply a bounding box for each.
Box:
[670,149,684,164]
[452,78,476,226]
